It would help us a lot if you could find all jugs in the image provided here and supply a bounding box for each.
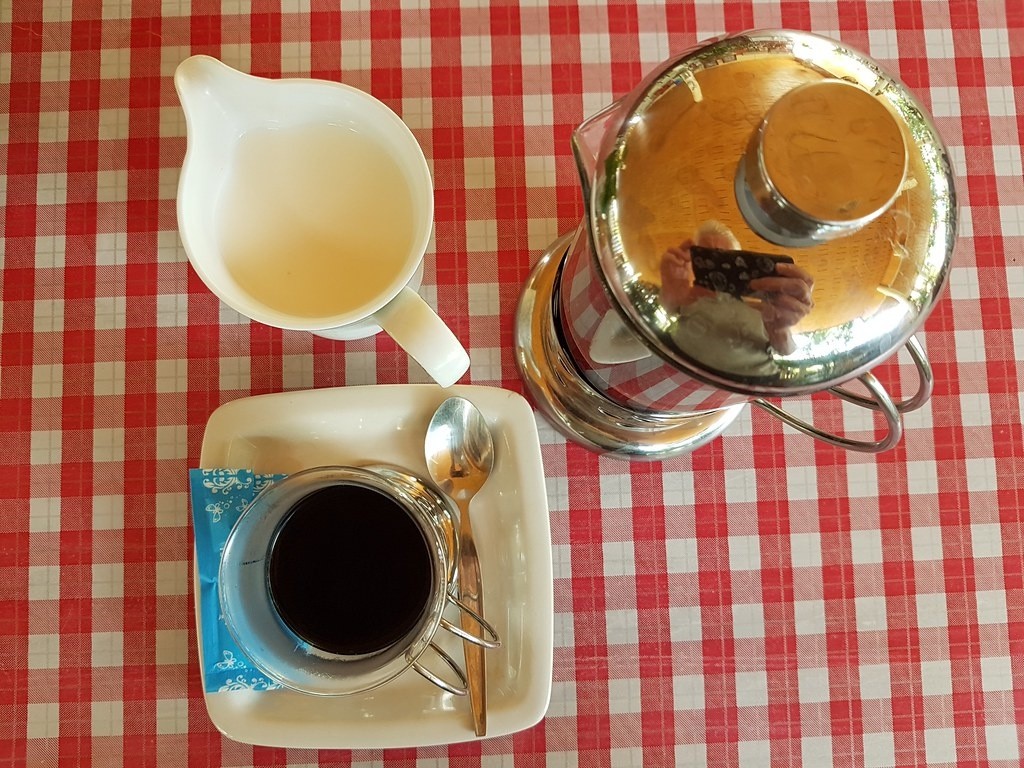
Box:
[513,25,959,462]
[172,53,473,389]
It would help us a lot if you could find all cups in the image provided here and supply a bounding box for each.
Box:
[216,461,503,697]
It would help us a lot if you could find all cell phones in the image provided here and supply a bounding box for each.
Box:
[689,245,793,298]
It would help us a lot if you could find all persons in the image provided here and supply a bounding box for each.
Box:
[658,219,817,382]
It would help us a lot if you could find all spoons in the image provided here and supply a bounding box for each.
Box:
[423,394,497,738]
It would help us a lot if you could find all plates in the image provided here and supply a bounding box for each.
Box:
[192,383,554,749]
